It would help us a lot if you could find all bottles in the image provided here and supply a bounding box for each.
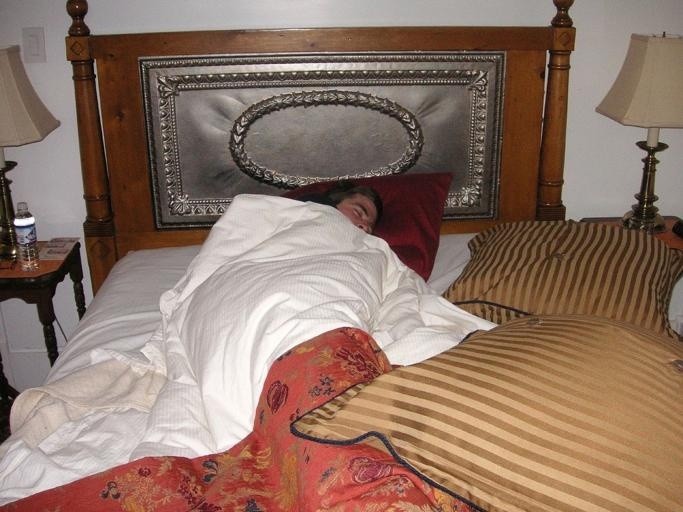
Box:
[14,201,41,274]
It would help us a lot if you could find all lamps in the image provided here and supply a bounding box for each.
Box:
[596,33,683,231]
[0,46,61,256]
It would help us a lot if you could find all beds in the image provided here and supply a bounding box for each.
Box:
[0,0,683,512]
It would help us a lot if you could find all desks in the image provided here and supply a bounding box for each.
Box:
[0,237,87,367]
[580,214,683,256]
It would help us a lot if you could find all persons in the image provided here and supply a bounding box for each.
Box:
[292,179,381,236]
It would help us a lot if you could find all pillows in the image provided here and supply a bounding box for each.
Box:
[439,217,682,340]
[280,169,454,286]
[289,313,682,509]
